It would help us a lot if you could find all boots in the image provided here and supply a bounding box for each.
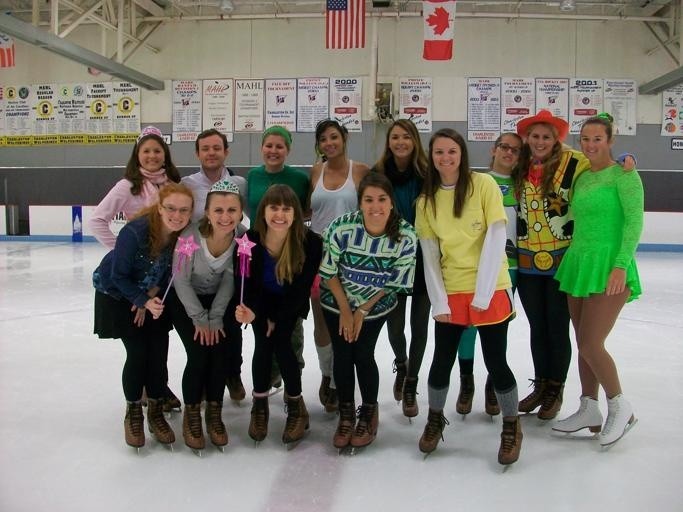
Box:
[485,374,501,415]
[393,357,407,401]
[552,396,603,433]
[498,416,523,464]
[125,373,244,449]
[402,376,418,417]
[456,375,474,414]
[600,394,634,445]
[418,407,445,452]
[250,371,379,448]
[538,378,564,420]
[518,376,546,412]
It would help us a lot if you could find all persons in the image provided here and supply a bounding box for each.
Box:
[233,184,324,449]
[317,170,418,457]
[372,119,431,422]
[517,110,646,449]
[242,124,310,393]
[88,125,194,455]
[170,129,247,455]
[415,127,524,466]
[306,118,370,419]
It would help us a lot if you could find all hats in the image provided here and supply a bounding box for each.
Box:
[517,109,569,141]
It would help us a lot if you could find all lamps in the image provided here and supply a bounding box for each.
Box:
[220,0,234,15]
[559,0,576,14]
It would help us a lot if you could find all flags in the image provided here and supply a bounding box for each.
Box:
[325,0,366,49]
[422,0,456,60]
[0,11,15,68]
